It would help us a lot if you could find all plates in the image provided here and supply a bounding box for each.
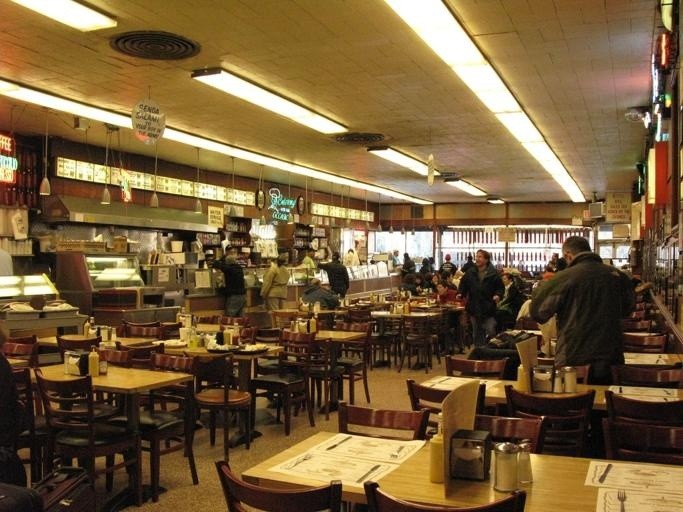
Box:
[154,340,182,350]
[208,345,272,354]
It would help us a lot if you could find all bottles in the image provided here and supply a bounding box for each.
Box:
[290,314,320,335]
[293,224,311,259]
[427,413,444,488]
[493,437,537,492]
[82,316,117,340]
[226,219,253,267]
[554,366,578,392]
[340,286,434,315]
[186,319,240,346]
[64,346,109,378]
[514,364,525,393]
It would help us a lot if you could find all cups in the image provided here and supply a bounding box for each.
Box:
[179,327,190,342]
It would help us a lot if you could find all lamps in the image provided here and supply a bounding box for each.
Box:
[10,0,118,33]
[191,68,349,133]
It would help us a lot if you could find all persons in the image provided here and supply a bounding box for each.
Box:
[211,245,247,344]
[529,235,638,386]
[437,249,524,344]
[392,250,437,297]
[545,253,558,276]
[344,249,354,266]
[260,245,349,328]
